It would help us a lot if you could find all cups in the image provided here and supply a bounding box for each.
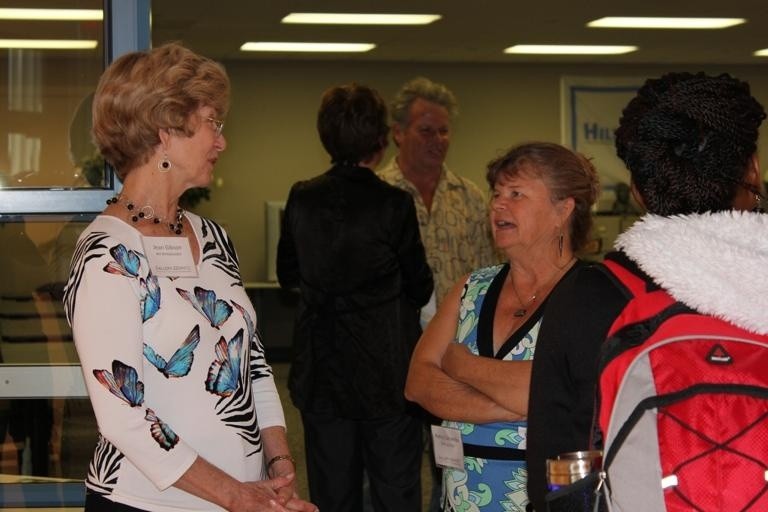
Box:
[546,449,604,490]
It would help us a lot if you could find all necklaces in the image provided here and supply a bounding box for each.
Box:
[104,196,185,236]
[508,255,575,317]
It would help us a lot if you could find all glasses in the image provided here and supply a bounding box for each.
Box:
[195,116,227,136]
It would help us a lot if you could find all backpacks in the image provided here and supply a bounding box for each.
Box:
[549,251,768,512]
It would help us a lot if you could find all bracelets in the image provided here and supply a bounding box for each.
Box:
[266,453,298,479]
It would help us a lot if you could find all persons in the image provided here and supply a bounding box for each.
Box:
[525,71,767,512]
[402,141,601,512]
[275,84,434,512]
[372,77,509,314]
[61,42,321,512]
